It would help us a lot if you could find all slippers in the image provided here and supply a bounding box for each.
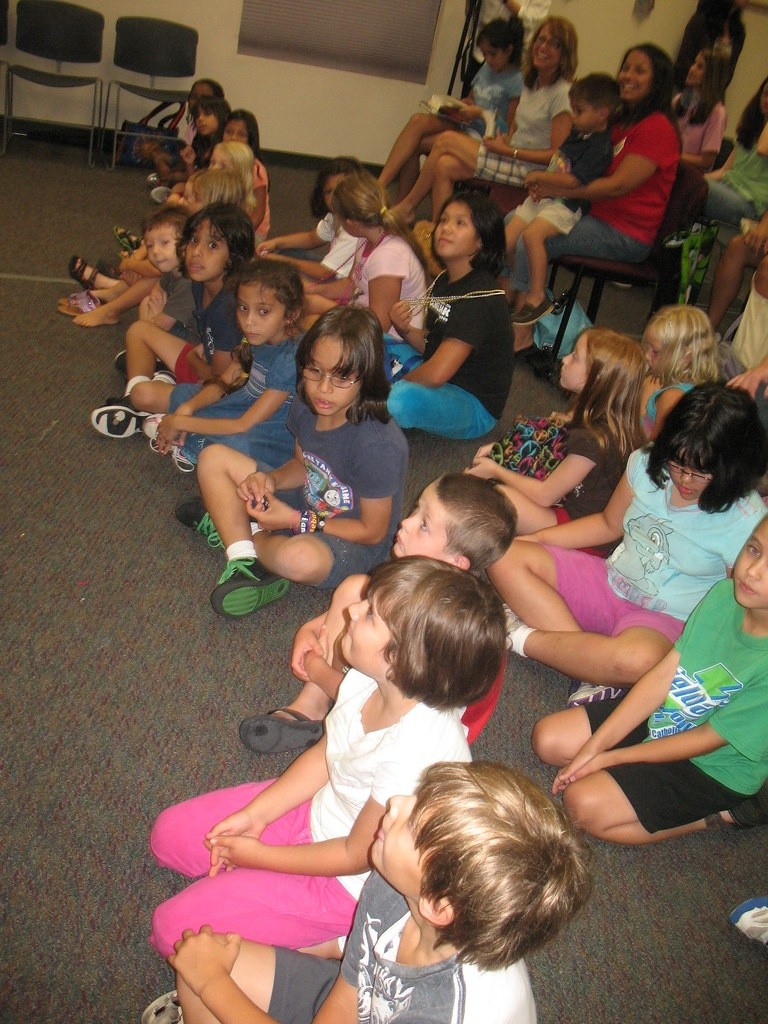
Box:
[57,290,100,315]
[239,708,326,754]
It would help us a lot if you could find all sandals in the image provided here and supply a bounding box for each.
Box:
[68,256,99,289]
[704,793,767,833]
[112,226,142,251]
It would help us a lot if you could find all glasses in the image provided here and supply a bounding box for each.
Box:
[664,462,714,484]
[301,367,363,388]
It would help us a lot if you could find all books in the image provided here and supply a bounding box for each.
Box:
[419,94,473,125]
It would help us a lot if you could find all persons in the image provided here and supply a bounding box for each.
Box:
[57,75,428,621]
[464,308,768,945]
[236,468,518,752]
[371,0,580,229]
[501,45,768,398]
[138,760,597,1024]
[377,188,514,440]
[145,556,505,961]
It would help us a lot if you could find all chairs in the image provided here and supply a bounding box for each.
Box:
[0,0,198,170]
[451,138,764,399]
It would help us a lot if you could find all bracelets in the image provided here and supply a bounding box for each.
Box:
[512,148,518,159]
[315,510,328,533]
[292,510,300,535]
[300,509,308,534]
[307,509,317,533]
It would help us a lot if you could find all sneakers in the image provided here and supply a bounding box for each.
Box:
[566,682,630,710]
[142,414,194,472]
[210,556,290,617]
[151,186,172,203]
[502,603,526,654]
[175,497,257,550]
[140,989,184,1024]
[90,394,152,438]
[146,172,161,187]
[729,896,768,945]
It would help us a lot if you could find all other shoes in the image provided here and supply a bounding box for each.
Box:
[513,294,555,326]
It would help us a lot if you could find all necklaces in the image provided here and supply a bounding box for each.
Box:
[301,230,388,305]
[399,268,506,317]
[326,233,336,252]
